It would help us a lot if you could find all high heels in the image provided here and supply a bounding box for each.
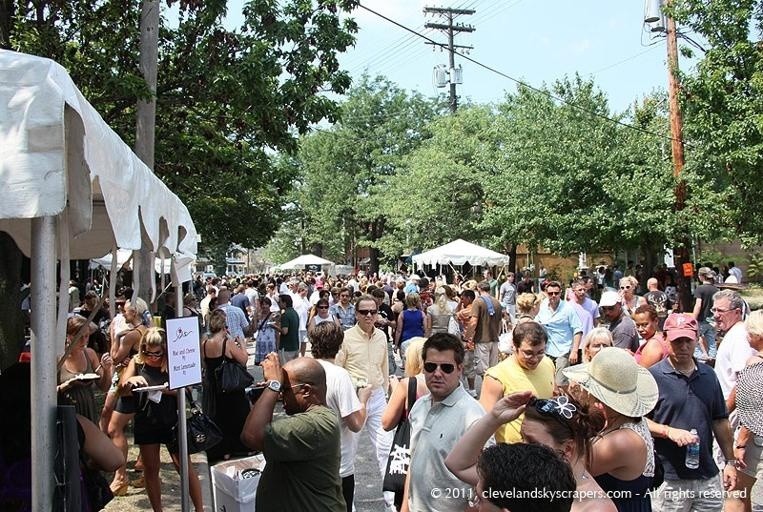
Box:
[109,478,144,496]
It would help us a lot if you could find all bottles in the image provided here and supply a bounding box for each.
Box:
[685,429,700,469]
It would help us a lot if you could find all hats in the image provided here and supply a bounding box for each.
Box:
[736,361,763,437]
[599,291,621,306]
[562,347,659,417]
[698,267,714,278]
[663,312,698,341]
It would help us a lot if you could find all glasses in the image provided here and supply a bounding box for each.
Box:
[424,363,454,373]
[143,351,163,357]
[548,292,559,296]
[522,351,544,359]
[526,396,572,430]
[358,310,377,315]
[319,306,328,309]
[620,286,630,290]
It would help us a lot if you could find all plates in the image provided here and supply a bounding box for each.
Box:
[267,321,276,325]
[77,375,101,381]
[132,385,165,392]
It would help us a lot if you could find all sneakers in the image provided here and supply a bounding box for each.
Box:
[465,388,477,397]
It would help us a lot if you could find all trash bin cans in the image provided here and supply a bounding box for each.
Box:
[210,454,266,512]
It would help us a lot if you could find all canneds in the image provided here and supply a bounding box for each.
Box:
[153,316,162,328]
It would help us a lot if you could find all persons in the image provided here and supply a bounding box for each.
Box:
[1,261,762,511]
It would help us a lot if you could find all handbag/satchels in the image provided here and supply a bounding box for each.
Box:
[383,422,410,493]
[172,410,223,454]
[215,360,253,394]
